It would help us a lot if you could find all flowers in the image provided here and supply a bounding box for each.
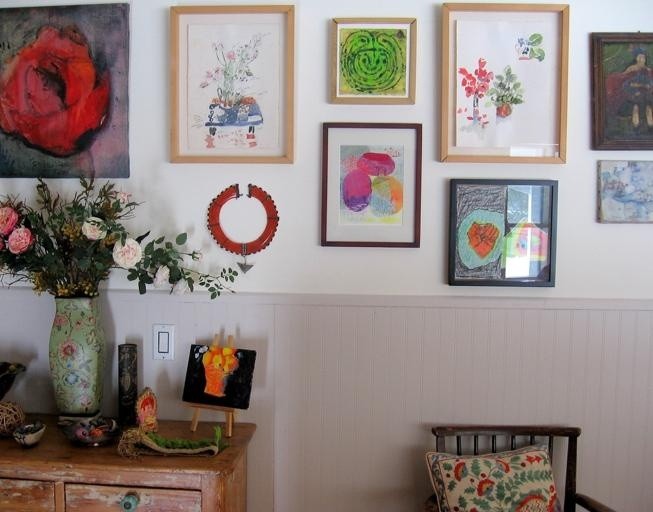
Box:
[0,176,238,301]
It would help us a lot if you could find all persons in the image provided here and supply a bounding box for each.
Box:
[620,52,652,130]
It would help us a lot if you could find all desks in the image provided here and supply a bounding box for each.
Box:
[0,413,257,511]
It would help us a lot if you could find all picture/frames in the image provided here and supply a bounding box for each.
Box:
[438,1,567,165]
[590,31,652,151]
[0,3,131,178]
[169,4,294,164]
[320,122,423,248]
[448,178,559,287]
[328,16,418,105]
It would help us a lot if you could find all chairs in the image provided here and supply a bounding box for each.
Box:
[425,424,616,511]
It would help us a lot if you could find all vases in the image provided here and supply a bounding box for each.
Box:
[48,300,107,412]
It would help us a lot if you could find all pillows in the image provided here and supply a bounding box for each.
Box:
[425,443,561,511]
